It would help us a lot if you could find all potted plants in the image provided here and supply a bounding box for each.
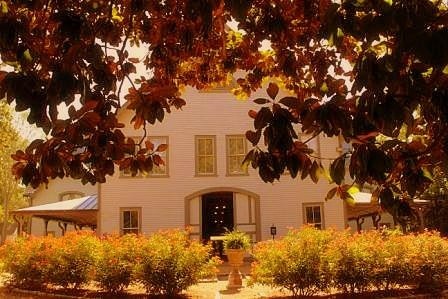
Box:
[222,226,252,288]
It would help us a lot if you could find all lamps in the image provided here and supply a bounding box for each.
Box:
[270,227,276,240]
